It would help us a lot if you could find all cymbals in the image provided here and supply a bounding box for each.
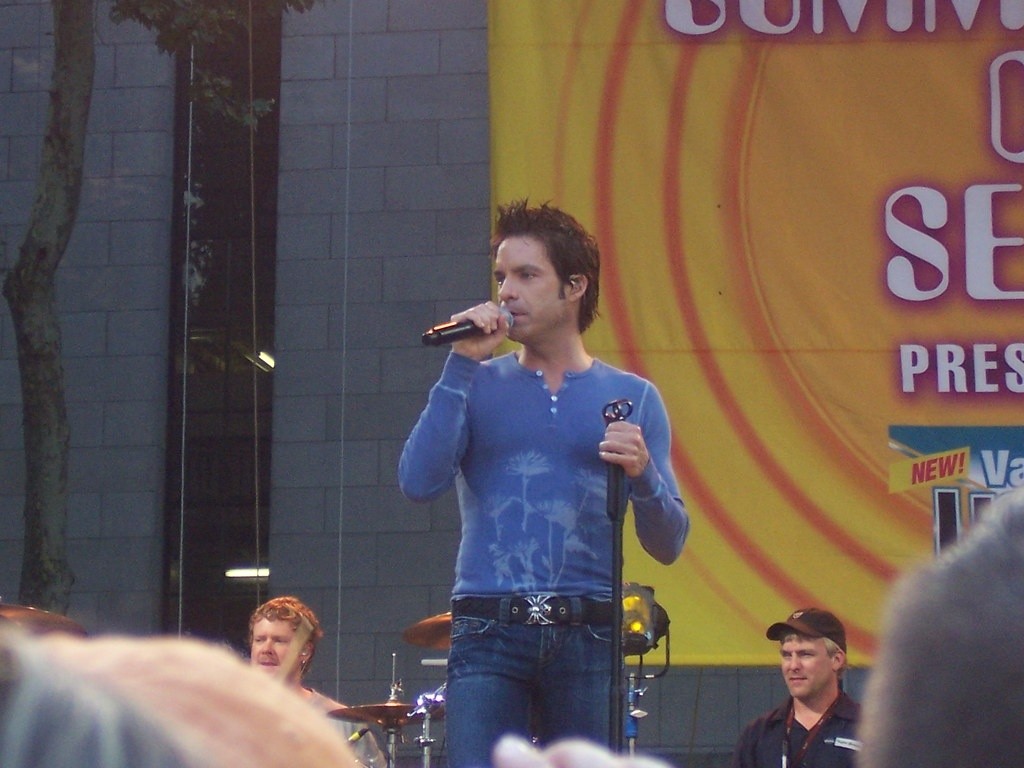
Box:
[325,700,445,723]
[402,612,453,650]
[0,599,95,638]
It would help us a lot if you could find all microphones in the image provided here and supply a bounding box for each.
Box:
[422,309,513,346]
[348,728,368,746]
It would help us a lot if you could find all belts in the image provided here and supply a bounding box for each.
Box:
[449,597,614,628]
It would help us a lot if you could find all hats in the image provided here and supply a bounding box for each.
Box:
[765,608,849,655]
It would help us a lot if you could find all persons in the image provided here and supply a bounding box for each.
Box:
[859,493,1023,768]
[249,597,387,768]
[0,624,670,768]
[397,196,691,768]
[733,608,862,768]
